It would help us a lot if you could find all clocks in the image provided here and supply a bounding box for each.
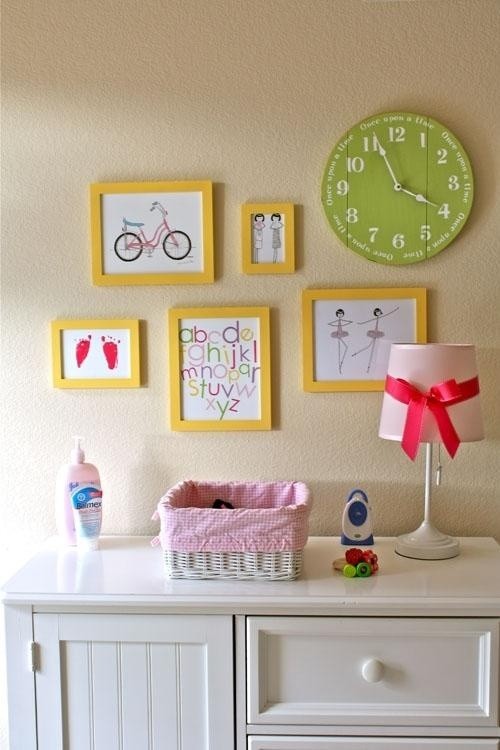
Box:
[321,112,477,268]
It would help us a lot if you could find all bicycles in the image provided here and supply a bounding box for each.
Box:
[114,201,191,262]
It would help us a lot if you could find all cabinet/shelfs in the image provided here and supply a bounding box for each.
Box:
[6,536,497,750]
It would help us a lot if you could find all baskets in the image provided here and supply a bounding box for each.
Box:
[159,481,311,581]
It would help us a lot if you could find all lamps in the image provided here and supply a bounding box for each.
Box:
[375,339,486,560]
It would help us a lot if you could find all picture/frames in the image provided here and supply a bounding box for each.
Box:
[301,285,424,394]
[169,306,271,432]
[243,205,296,274]
[49,318,142,390]
[88,179,215,285]
